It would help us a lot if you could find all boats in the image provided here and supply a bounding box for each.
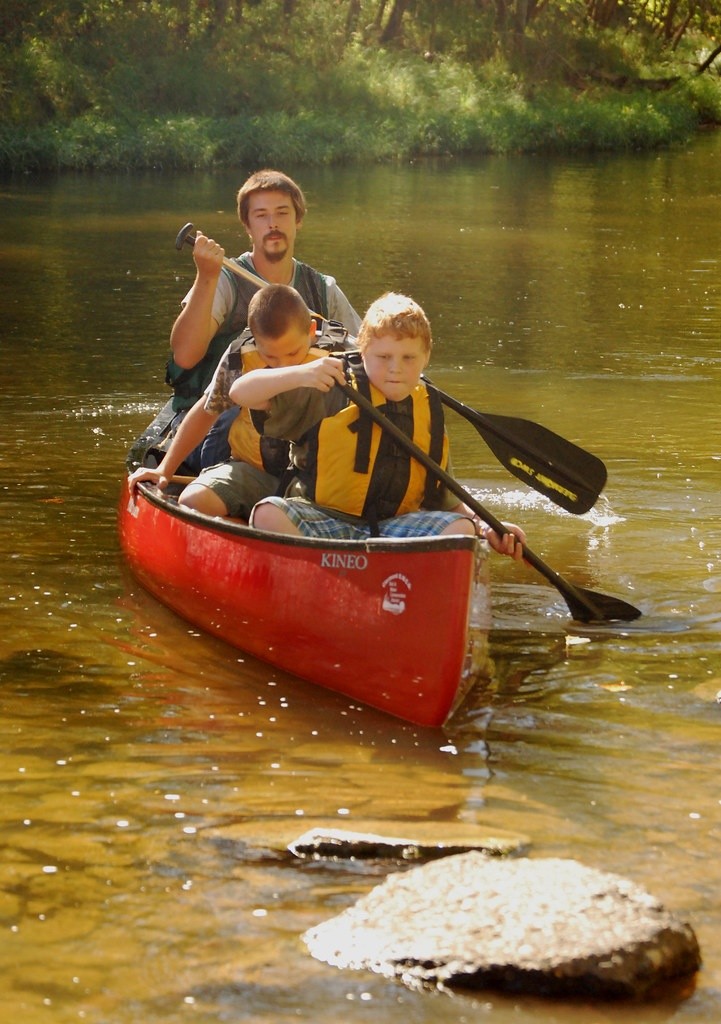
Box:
[117,464,492,730]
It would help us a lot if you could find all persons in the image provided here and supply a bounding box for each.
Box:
[227,292,526,564]
[126,284,344,516]
[167,169,364,475]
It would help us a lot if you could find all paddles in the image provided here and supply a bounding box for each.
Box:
[180,217,609,519]
[323,374,642,632]
